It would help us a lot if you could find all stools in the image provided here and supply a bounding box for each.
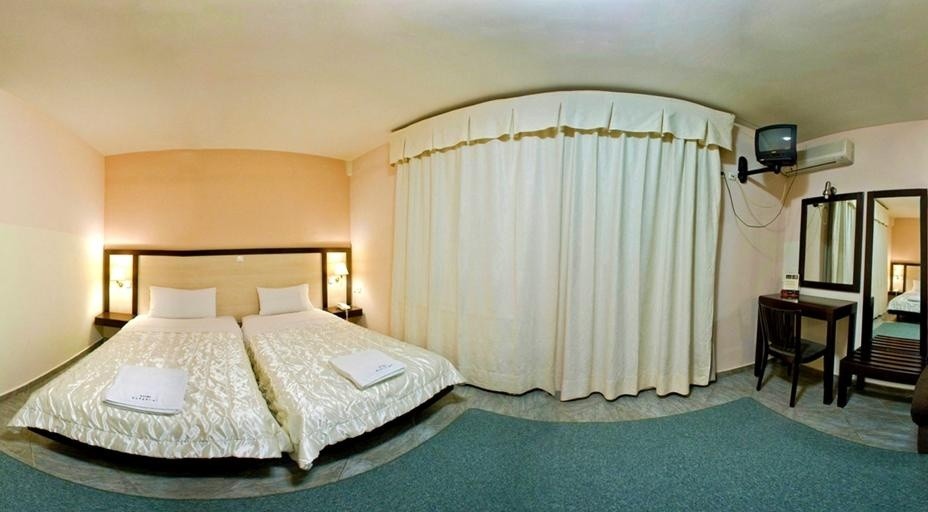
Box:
[872,330,921,354]
[836,347,921,408]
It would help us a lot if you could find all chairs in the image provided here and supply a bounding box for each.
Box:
[756,306,821,407]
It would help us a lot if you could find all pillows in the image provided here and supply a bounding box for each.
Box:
[256,284,313,315]
[148,287,215,318]
[912,279,920,291]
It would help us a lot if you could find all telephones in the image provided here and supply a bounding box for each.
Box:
[337,302,351,311]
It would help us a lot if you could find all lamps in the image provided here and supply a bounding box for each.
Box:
[334,264,349,284]
[823,180,836,200]
[112,265,125,288]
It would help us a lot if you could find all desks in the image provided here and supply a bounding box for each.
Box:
[755,289,859,405]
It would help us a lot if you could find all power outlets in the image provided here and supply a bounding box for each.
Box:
[720,169,726,179]
[727,172,736,181]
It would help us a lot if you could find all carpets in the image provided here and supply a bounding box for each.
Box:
[1,395,925,511]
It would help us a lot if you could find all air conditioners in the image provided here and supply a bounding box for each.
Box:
[777,140,853,175]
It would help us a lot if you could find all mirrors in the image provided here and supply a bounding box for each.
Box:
[799,191,864,294]
[861,185,925,401]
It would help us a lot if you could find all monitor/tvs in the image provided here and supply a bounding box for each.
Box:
[755,124,797,167]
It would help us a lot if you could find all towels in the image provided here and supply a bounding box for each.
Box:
[907,296,920,301]
[102,365,188,417]
[327,348,408,390]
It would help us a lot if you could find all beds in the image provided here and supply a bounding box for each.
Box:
[8,285,294,475]
[241,284,463,473]
[888,281,921,320]
